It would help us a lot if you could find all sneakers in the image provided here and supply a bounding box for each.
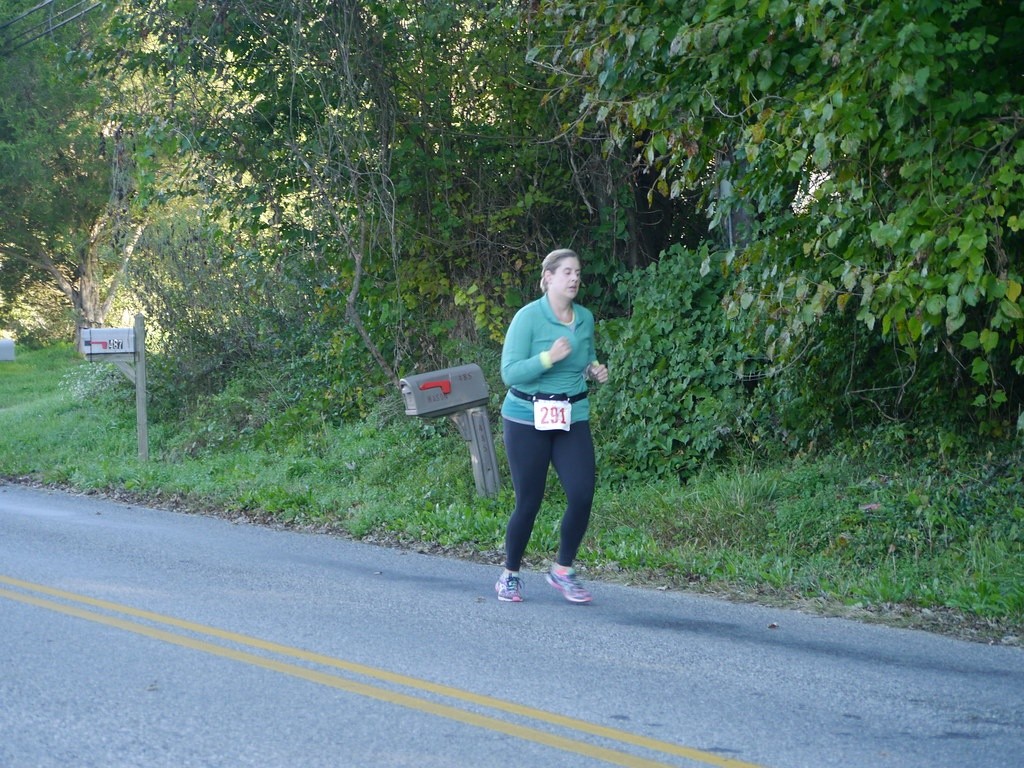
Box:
[495,572,525,602]
[545,564,593,602]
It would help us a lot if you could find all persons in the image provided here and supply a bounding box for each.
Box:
[494,249,609,602]
